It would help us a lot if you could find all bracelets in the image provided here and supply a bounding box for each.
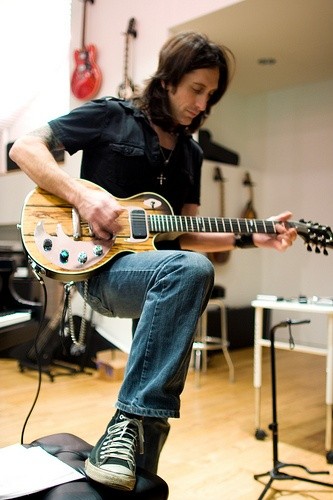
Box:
[235,233,257,248]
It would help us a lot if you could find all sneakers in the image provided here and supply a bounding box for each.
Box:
[85,408,145,492]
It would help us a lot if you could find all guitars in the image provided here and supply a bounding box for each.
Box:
[116,19,139,101]
[18,179,333,281]
[69,0,104,102]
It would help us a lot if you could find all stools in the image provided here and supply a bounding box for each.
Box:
[23,433,168,500]
[190,286,235,387]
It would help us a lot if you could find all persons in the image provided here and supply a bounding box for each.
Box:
[9,34,297,491]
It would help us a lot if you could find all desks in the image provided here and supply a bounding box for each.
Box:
[250,300,333,465]
